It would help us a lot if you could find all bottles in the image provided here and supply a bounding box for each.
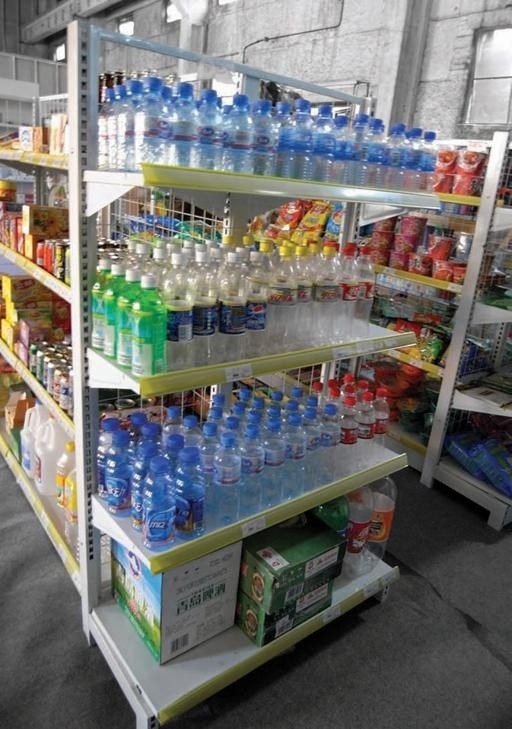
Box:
[91,234,376,377]
[95,372,392,552]
[52,441,80,568]
[313,477,397,577]
[97,76,438,194]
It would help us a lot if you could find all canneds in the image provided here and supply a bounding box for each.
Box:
[37,239,70,286]
[28,337,74,420]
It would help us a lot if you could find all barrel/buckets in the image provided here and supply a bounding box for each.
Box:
[18,400,79,548]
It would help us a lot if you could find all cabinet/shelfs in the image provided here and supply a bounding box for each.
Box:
[1,19,511,728]
